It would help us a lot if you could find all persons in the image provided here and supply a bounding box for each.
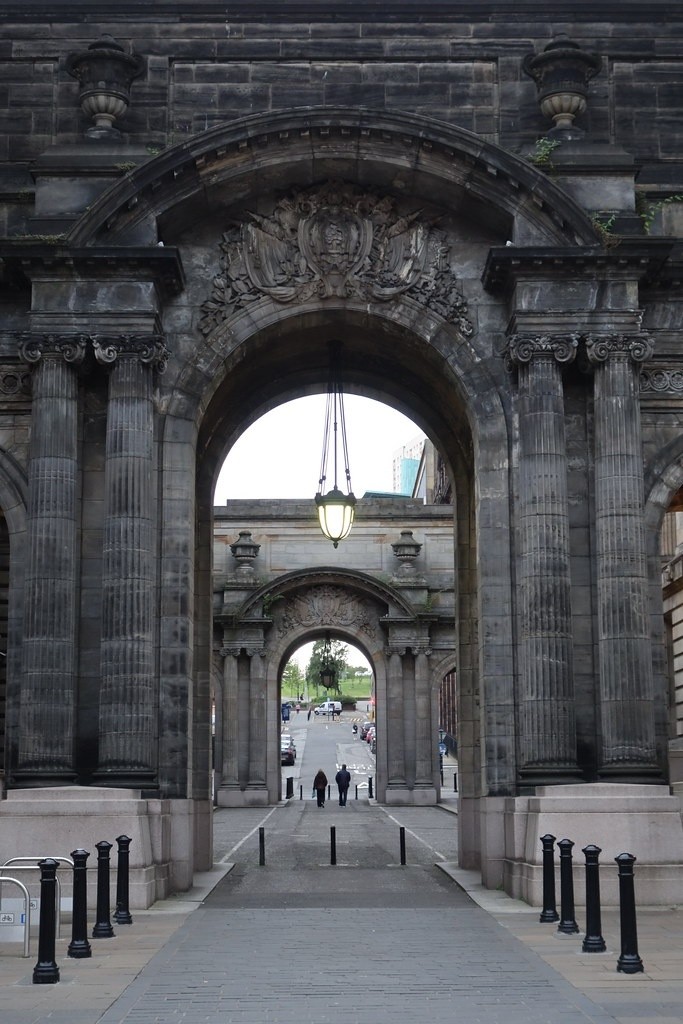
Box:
[313,768,328,808]
[296,703,300,714]
[352,723,358,741]
[336,765,350,807]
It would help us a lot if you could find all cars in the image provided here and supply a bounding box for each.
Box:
[369,733,377,754]
[280,740,295,766]
[281,734,296,758]
[359,721,375,741]
[365,727,376,743]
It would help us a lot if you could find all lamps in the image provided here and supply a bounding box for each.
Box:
[320,629,336,690]
[314,340,357,548]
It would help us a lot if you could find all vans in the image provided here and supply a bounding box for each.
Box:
[314,702,342,716]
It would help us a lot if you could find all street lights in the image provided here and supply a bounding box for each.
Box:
[438,729,445,787]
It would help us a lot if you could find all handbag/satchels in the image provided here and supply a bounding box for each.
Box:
[311,790,315,798]
[352,728,356,732]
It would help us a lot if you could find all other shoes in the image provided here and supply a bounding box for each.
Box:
[318,806,320,808]
[321,803,324,808]
[338,805,346,808]
